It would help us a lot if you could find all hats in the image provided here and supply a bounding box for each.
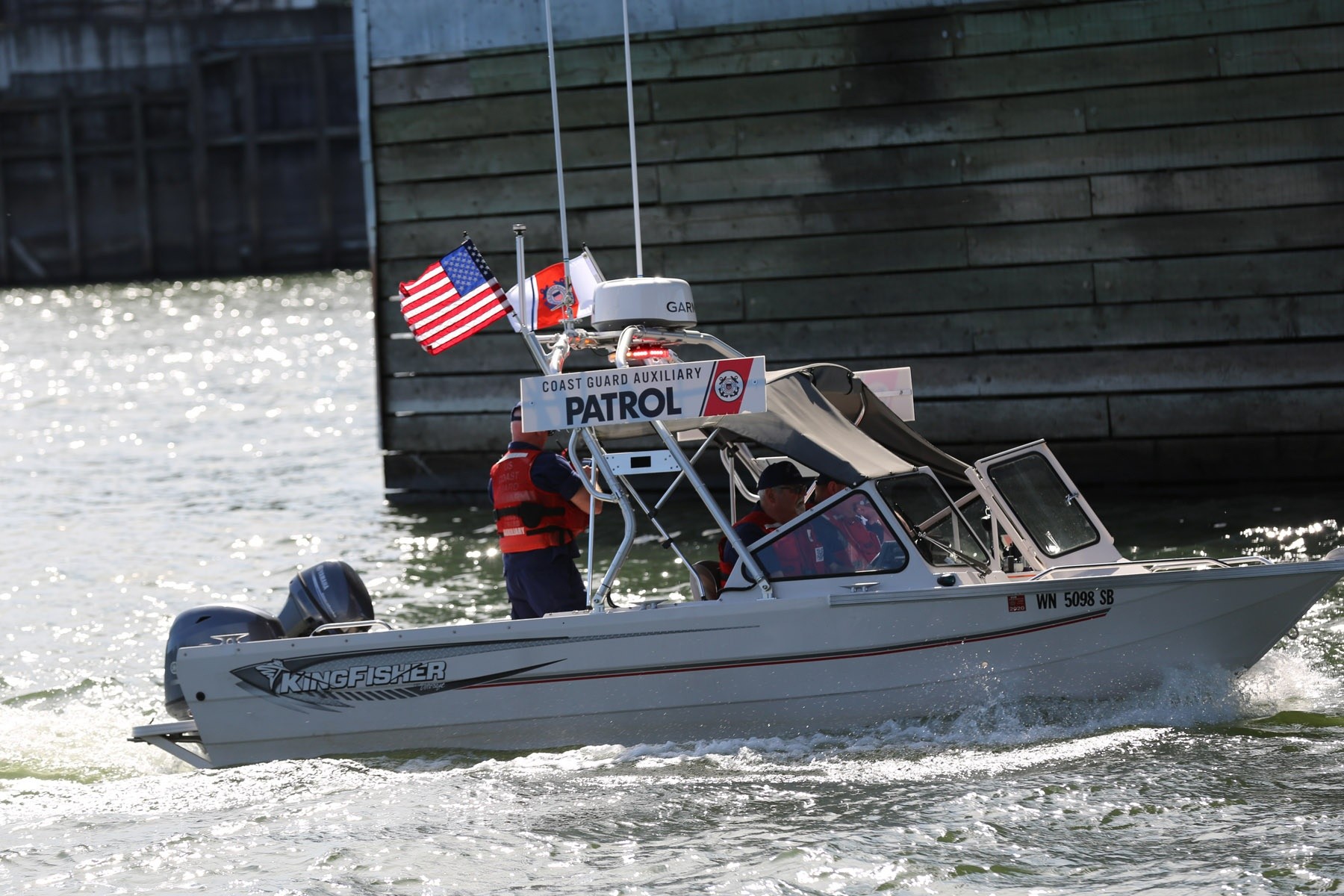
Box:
[816,474,842,484]
[511,402,556,437]
[756,461,806,491]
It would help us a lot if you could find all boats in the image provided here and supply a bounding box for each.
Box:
[127,0,1344,772]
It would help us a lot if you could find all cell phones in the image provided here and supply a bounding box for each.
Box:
[581,457,592,467]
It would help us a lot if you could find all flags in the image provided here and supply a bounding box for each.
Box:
[397,240,513,358]
[503,254,601,333]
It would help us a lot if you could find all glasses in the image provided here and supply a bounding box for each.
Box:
[779,484,808,496]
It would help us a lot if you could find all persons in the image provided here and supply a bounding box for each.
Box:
[806,469,910,574]
[491,400,603,620]
[720,461,823,594]
